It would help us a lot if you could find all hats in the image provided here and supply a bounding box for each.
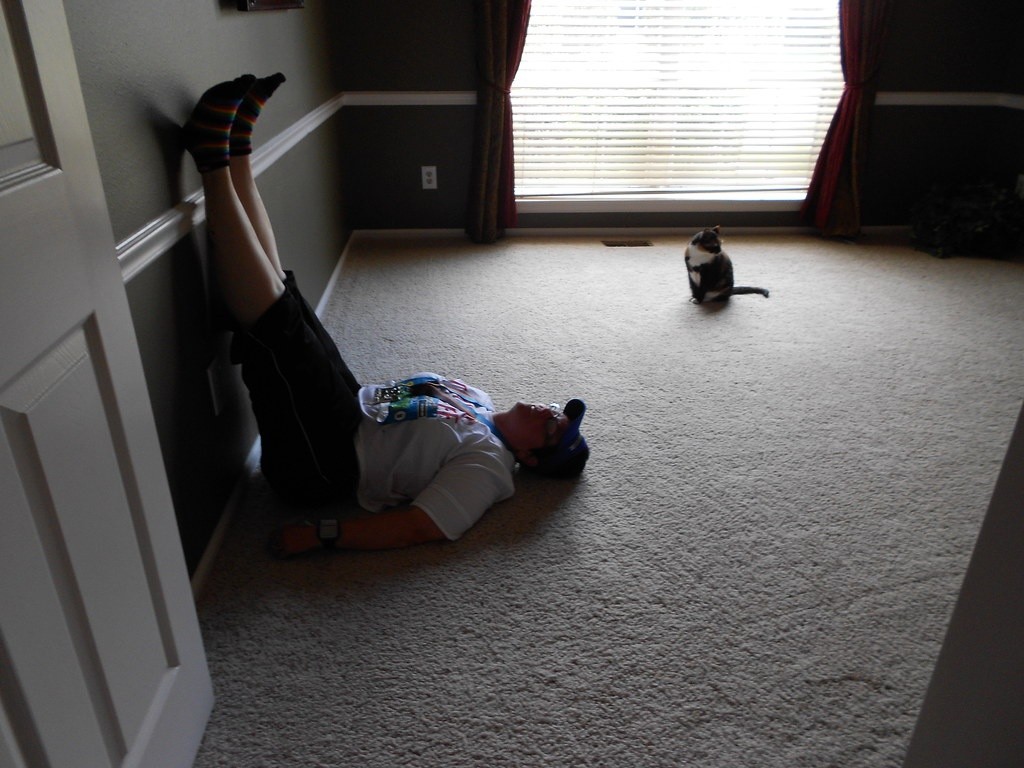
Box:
[537,400,590,478]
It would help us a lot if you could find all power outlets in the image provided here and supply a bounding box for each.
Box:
[421,166,437,189]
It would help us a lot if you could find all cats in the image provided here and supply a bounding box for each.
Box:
[684,225,768,304]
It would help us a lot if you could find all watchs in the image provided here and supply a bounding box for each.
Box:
[317,516,342,556]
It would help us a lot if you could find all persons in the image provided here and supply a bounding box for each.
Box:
[174,71,590,557]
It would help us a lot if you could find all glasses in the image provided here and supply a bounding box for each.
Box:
[544,402,560,447]
